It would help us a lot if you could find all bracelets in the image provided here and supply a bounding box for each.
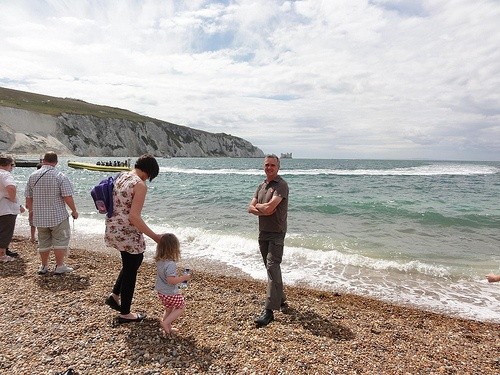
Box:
[20,205,22,207]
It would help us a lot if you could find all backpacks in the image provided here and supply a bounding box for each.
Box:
[90,172,121,218]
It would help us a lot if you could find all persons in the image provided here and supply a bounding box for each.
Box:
[24,152,79,275]
[247,154,290,324]
[0,153,25,263]
[27,164,43,240]
[39,159,42,163]
[154,234,189,334]
[96,158,131,168]
[91,156,163,323]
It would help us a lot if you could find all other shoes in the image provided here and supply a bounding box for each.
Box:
[6,249,19,257]
[119,312,147,323]
[0,256,15,262]
[105,296,122,311]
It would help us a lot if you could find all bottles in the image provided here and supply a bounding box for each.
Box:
[181,270,187,287]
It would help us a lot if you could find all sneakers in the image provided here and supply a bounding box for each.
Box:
[56,265,73,274]
[253,310,273,325]
[38,266,48,274]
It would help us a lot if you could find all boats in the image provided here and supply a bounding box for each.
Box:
[280,152,293,159]
[67,159,131,172]
[14,158,43,167]
